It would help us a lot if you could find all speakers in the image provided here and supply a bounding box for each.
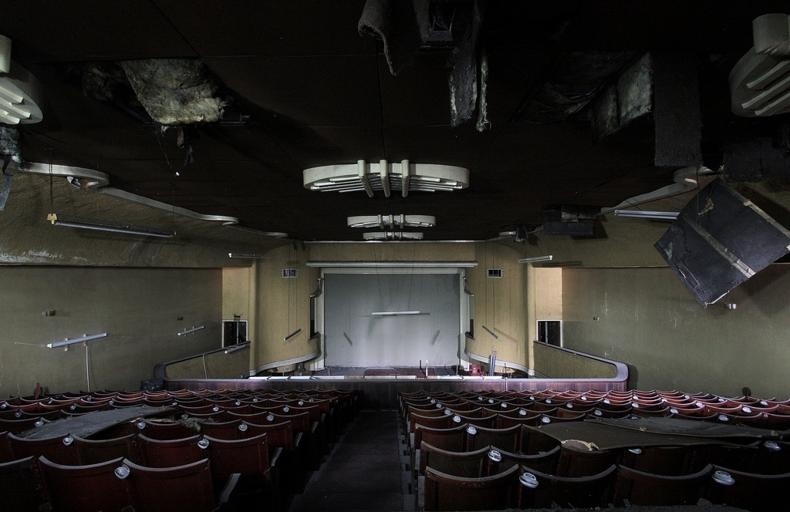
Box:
[653,177,790,306]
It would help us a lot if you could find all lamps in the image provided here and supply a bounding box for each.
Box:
[43,163,178,239]
[518,236,553,265]
[602,195,680,221]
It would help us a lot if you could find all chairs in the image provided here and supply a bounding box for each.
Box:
[0,380,364,512]
[393,385,790,512]
